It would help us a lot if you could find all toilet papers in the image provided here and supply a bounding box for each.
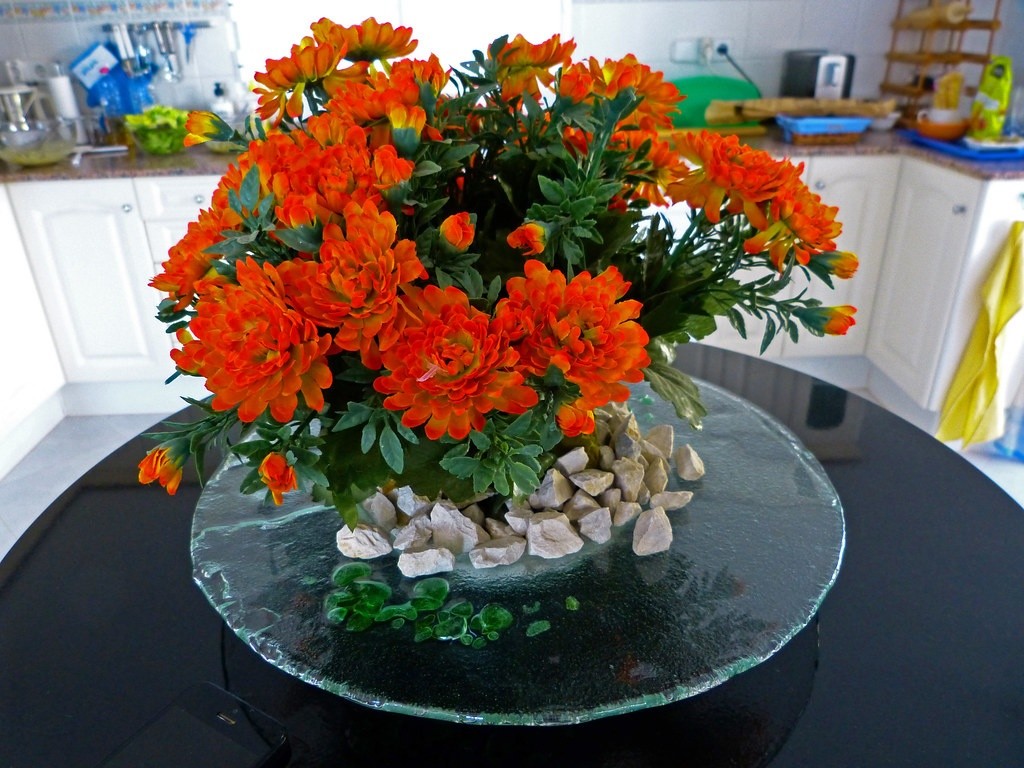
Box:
[47,75,88,146]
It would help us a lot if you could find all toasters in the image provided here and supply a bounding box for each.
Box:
[778,50,857,99]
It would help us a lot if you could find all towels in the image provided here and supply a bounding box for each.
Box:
[932,221,1024,454]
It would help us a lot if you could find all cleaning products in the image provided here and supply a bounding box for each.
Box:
[211,80,233,122]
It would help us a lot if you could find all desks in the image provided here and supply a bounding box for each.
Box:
[0,340,1023,768]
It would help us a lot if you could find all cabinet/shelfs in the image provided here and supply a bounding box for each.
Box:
[7,175,221,382]
[865,160,1024,412]
[628,156,901,358]
[1,186,65,437]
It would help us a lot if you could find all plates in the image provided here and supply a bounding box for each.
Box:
[960,134,1024,152]
[72,144,128,155]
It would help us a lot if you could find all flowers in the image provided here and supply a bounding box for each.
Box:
[137,17,861,532]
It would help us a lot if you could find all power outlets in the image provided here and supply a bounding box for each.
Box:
[671,39,731,64]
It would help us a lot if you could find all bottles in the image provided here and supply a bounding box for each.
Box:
[46,60,87,145]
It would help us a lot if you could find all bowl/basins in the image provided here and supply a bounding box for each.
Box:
[0,119,80,167]
[916,121,969,141]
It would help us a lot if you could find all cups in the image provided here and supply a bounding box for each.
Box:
[919,109,963,124]
